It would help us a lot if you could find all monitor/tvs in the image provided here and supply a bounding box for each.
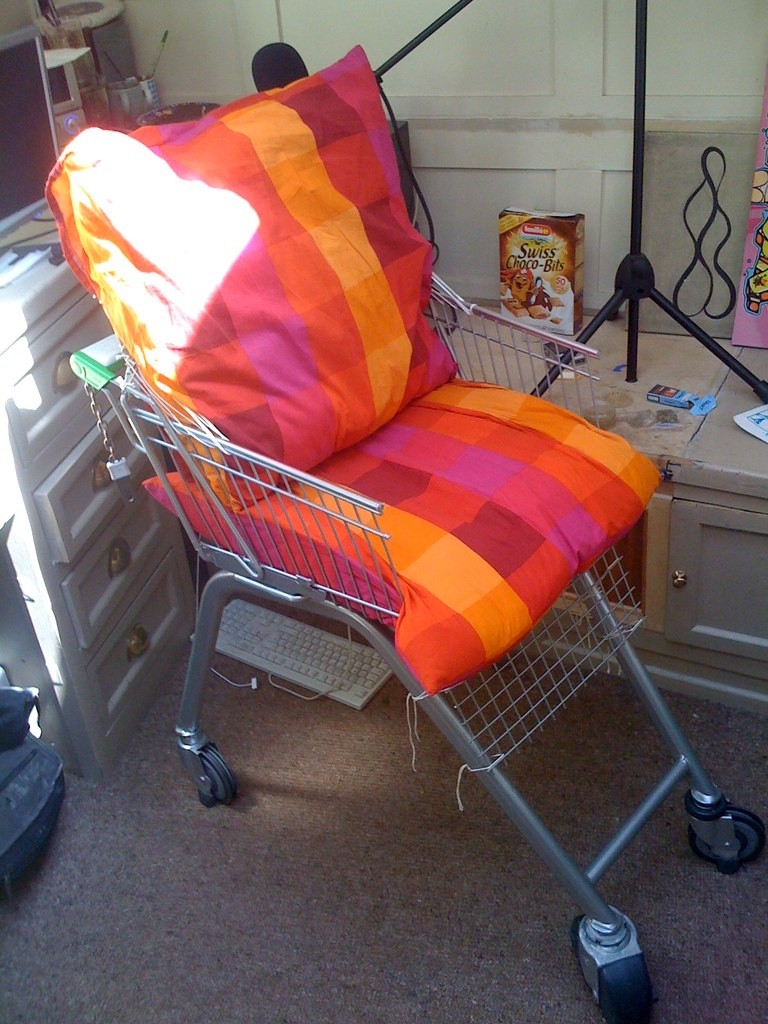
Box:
[0,25,62,286]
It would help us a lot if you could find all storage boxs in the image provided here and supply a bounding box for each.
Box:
[498,206,586,337]
[544,343,586,366]
[647,383,716,417]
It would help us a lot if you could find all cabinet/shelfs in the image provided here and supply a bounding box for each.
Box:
[0,249,201,784]
[421,300,768,718]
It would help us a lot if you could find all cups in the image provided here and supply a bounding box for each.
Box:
[125,74,160,109]
[107,84,146,130]
[576,363,617,430]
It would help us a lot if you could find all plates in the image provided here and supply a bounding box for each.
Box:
[136,102,220,127]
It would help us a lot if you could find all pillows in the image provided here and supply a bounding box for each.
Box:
[45,44,459,517]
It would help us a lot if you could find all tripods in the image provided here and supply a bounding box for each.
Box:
[531,0,768,407]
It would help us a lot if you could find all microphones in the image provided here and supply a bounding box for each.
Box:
[252,43,308,94]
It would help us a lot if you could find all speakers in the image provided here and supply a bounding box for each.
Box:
[88,18,135,86]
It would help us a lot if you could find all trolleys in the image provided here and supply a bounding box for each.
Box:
[69,271,764,1024]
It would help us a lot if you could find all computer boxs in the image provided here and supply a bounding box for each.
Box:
[47,61,86,157]
